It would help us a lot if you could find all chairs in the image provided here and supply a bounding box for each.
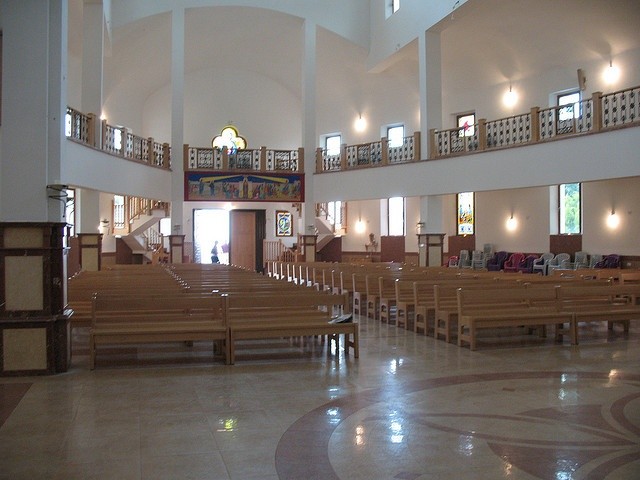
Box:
[448,243,623,275]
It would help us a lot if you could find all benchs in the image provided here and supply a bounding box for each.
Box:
[66,263,359,370]
[266,261,639,350]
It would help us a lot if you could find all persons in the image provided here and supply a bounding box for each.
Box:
[210,241,220,264]
